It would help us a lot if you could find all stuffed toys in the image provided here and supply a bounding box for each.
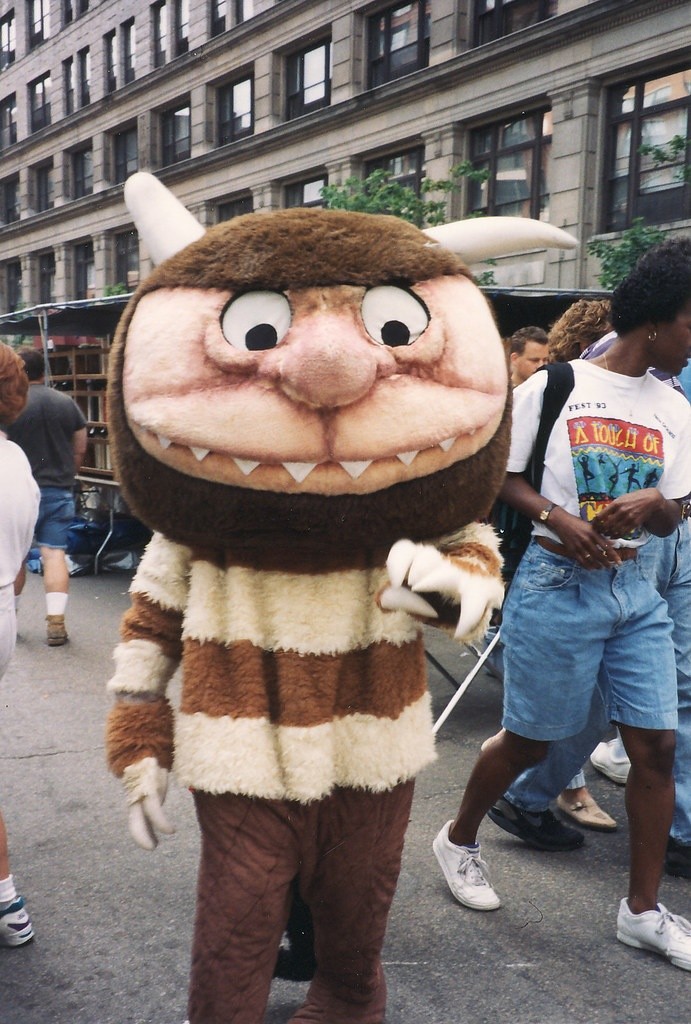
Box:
[104,171,580,1024]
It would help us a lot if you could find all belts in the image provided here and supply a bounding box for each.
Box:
[534,536,638,561]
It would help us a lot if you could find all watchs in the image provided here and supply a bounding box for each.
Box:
[540,503,557,523]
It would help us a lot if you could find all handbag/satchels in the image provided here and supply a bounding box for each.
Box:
[485,364,574,583]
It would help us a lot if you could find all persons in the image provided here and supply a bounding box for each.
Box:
[6,347,88,646]
[432,237,691,972]
[0,340,41,947]
[479,301,691,877]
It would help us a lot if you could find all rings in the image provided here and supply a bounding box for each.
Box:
[585,554,591,559]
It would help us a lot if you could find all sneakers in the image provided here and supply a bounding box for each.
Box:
[0,897,34,947]
[616,896,691,972]
[433,820,502,911]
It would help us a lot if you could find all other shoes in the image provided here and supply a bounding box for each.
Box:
[664,835,691,879]
[487,796,585,853]
[557,794,617,833]
[589,737,631,783]
[45,615,68,647]
